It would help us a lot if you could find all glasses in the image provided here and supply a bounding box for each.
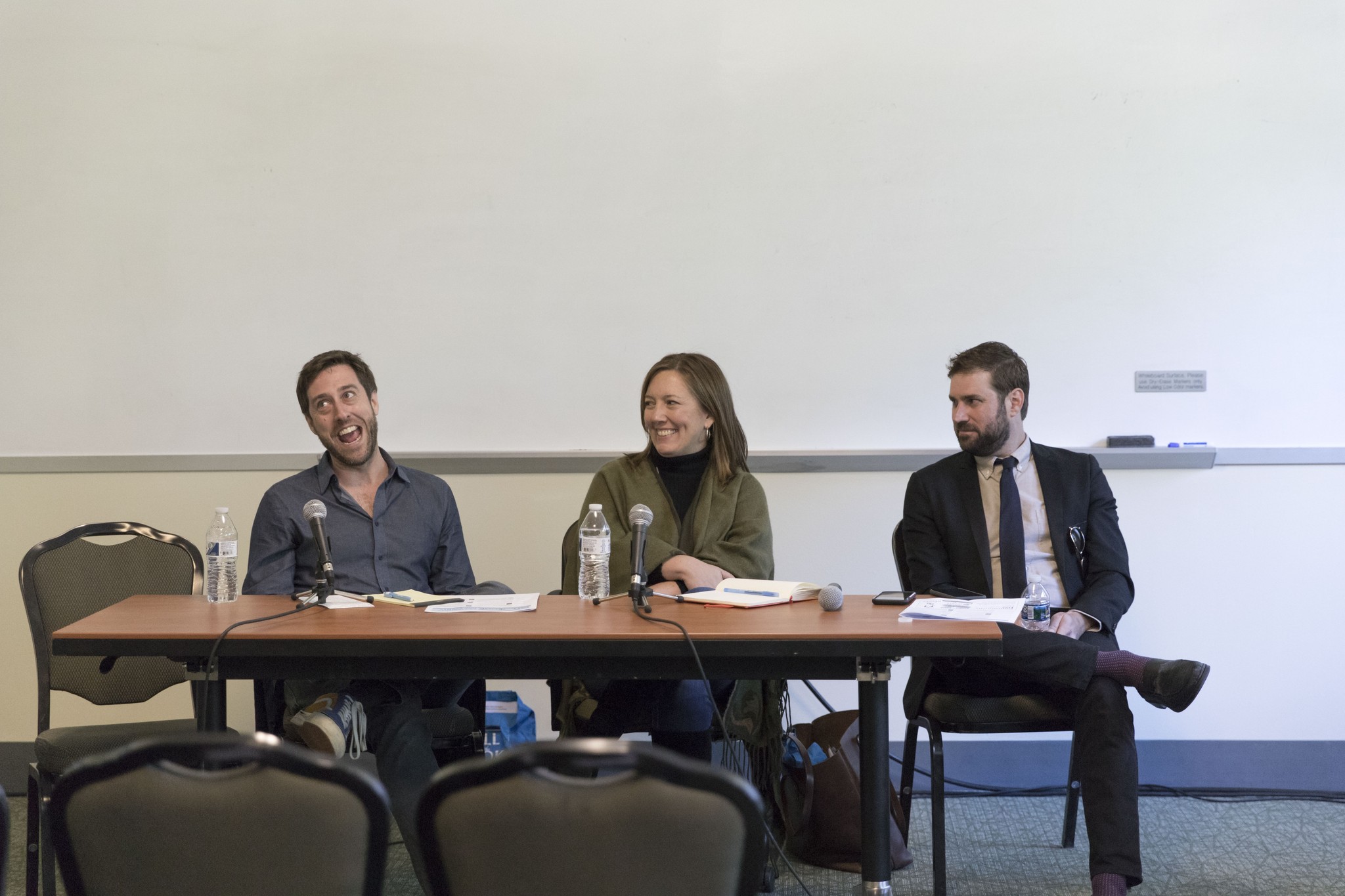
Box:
[1069,527,1088,579]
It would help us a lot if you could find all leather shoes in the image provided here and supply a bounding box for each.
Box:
[1138,658,1210,713]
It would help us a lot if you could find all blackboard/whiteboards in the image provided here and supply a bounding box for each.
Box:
[2,1,1345,477]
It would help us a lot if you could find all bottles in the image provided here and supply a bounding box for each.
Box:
[206,507,238,603]
[578,502,611,599]
[1021,577,1049,634]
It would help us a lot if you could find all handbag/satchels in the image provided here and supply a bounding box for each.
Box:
[483,690,536,758]
[775,709,914,872]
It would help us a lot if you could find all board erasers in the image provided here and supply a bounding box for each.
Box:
[1106,435,1154,447]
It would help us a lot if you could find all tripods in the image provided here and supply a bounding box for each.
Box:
[589,587,689,616]
[290,586,377,610]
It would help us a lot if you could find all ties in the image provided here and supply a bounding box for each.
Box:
[994,457,1027,598]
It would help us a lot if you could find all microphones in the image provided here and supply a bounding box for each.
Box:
[301,497,339,592]
[818,581,843,611]
[624,501,656,604]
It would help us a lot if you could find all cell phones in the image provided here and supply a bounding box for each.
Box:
[929,584,987,601]
[871,590,918,607]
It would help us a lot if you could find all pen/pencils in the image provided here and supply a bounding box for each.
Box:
[724,588,779,597]
[383,592,414,601]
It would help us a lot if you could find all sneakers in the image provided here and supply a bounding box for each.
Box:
[290,693,367,759]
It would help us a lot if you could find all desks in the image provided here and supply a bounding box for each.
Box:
[54,596,1003,896]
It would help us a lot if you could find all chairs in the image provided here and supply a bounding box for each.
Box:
[20,519,1080,896]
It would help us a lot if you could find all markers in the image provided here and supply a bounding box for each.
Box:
[1168,442,1207,448]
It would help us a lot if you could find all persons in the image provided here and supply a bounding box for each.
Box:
[241,349,517,896]
[902,341,1211,896]
[553,352,776,781]
